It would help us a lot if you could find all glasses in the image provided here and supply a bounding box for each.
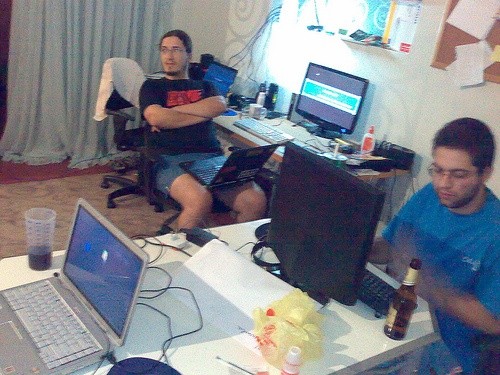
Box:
[426,166,479,181]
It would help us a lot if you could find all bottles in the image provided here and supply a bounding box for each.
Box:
[240,99,250,120]
[384,258,422,340]
[361,125,375,154]
[280,346,301,375]
[254,82,279,110]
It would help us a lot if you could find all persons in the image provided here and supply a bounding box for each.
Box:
[369,117,500,375]
[138,29,267,229]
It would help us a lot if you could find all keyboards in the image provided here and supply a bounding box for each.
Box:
[356,269,420,320]
[233,115,295,145]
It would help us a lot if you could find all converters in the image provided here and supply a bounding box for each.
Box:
[186,226,219,246]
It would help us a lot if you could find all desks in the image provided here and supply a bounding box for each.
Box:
[0,214,441,375]
[214,111,413,181]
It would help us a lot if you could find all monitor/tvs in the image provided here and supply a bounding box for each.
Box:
[265,138,386,305]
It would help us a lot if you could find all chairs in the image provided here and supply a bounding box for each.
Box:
[90,56,181,213]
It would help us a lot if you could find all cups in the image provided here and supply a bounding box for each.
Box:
[250,104,267,120]
[24,207,56,272]
[324,153,348,169]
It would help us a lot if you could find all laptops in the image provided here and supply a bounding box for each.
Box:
[179,142,279,189]
[203,60,238,98]
[293,62,370,138]
[0,197,149,375]
